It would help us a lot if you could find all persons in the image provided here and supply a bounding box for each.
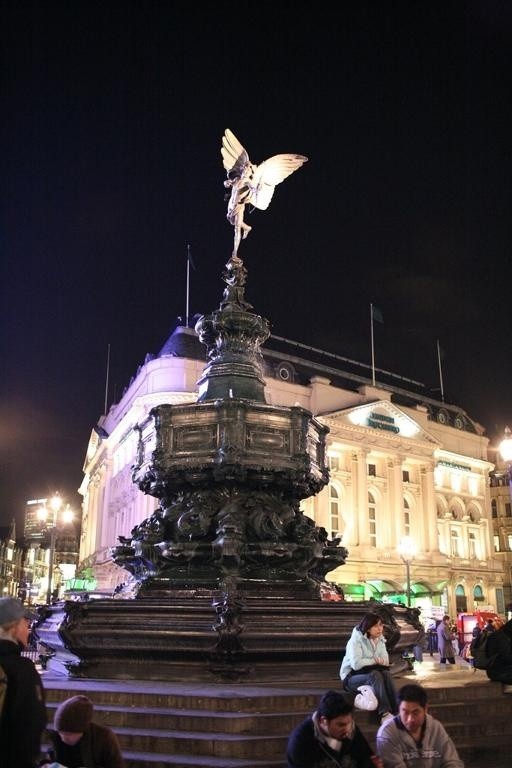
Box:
[41,695,127,768]
[288,688,383,767]
[338,613,402,726]
[411,616,512,685]
[219,160,259,261]
[0,595,50,768]
[377,684,464,768]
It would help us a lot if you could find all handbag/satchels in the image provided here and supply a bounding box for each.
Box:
[470,631,498,670]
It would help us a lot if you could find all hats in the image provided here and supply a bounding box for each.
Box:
[0,598,39,626]
[54,696,92,732]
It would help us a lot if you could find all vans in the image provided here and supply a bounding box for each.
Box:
[455,612,506,648]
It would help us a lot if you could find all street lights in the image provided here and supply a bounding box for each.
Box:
[37,490,75,603]
[395,535,420,609]
[498,423,511,478]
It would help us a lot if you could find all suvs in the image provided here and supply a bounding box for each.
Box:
[64,589,115,603]
